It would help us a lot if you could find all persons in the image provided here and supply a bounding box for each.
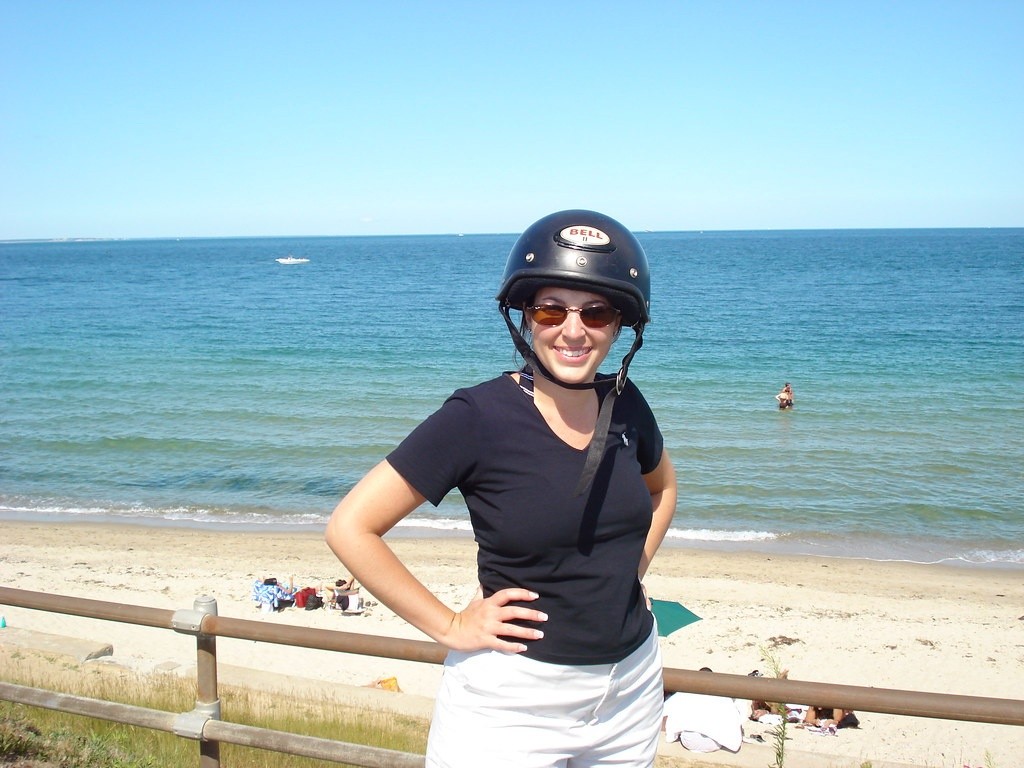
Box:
[694,668,857,735]
[256,574,355,612]
[775,382,794,410]
[324,209,678,768]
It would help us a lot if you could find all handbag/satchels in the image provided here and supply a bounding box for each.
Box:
[305,594,325,610]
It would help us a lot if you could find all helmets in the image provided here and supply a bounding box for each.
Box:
[495,209,650,327]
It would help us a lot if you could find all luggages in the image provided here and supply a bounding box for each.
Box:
[296,587,316,607]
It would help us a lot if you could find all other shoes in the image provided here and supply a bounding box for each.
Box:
[750,734,766,743]
[764,729,794,740]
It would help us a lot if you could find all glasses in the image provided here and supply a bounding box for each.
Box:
[523,300,620,328]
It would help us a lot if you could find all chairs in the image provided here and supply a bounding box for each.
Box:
[251,581,298,607]
[330,588,360,610]
